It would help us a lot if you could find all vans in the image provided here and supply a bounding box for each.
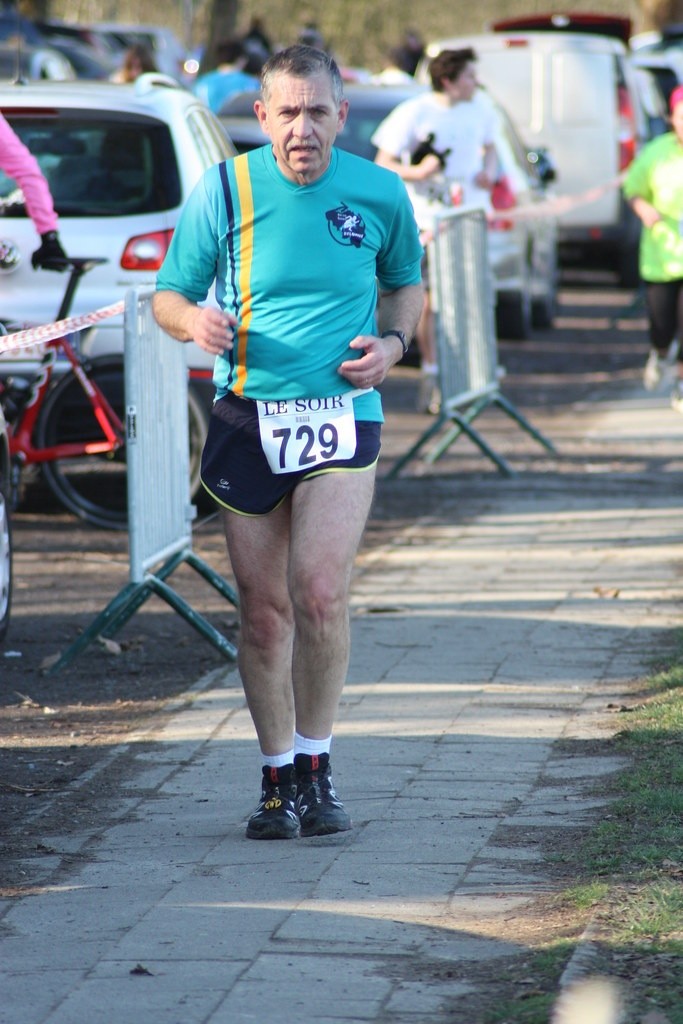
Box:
[414,13,683,288]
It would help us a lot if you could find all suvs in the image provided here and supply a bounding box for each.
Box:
[1,74,240,424]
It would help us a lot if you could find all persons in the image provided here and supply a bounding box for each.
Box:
[0,110,69,271]
[98,45,155,201]
[625,87,683,413]
[242,15,271,76]
[298,21,321,49]
[196,39,260,114]
[369,48,499,413]
[152,45,426,838]
[401,34,424,75]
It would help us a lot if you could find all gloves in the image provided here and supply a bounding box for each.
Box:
[32,232,69,271]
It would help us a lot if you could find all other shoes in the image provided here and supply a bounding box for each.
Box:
[643,346,667,390]
[418,374,440,415]
[671,376,683,412]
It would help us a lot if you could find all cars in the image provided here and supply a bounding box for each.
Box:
[475,90,560,338]
[39,15,410,166]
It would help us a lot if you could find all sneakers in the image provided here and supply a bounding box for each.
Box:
[293,752,351,837]
[246,764,300,838]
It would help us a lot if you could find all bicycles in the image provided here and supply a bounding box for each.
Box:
[1,255,210,533]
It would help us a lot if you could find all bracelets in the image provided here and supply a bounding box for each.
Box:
[381,330,408,353]
